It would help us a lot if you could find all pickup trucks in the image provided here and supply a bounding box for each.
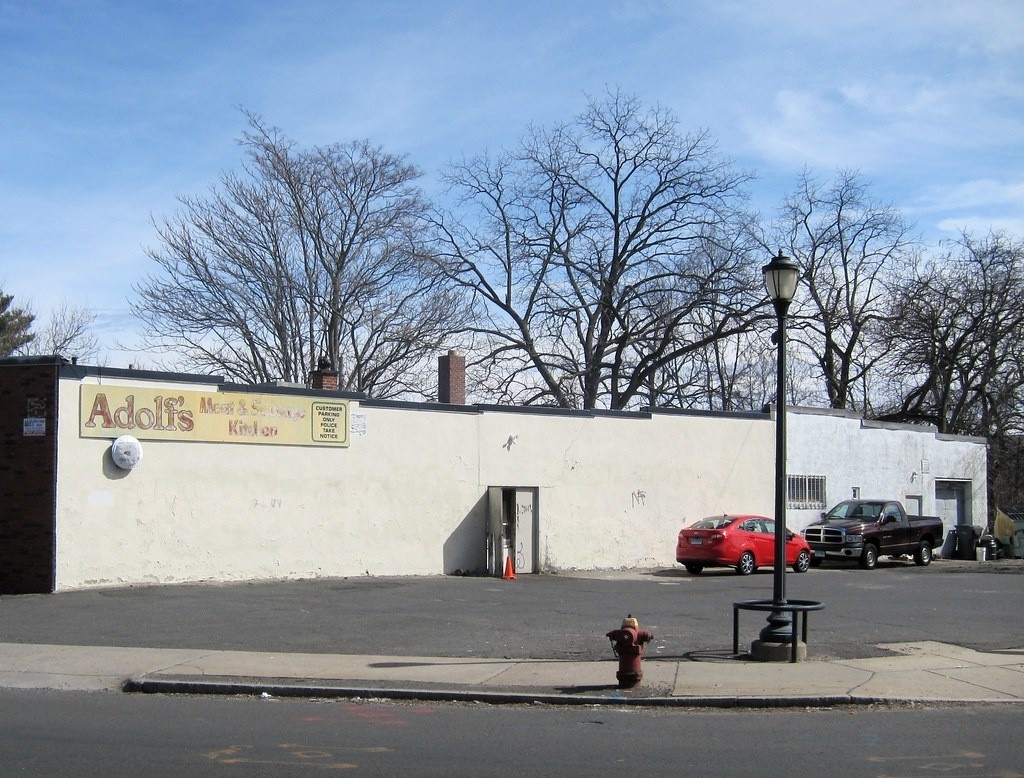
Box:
[800,499,944,571]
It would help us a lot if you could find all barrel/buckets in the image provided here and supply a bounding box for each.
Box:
[976,547,986,561]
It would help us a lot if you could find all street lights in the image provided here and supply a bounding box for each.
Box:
[748,247,808,665]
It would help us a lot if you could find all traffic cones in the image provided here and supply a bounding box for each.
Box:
[501,556,518,580]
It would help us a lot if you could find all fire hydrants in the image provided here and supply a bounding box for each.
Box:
[603,613,655,689]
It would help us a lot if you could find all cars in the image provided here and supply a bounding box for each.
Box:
[676,514,811,576]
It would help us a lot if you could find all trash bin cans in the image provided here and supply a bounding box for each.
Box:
[954,524,983,560]
[975,547,987,562]
